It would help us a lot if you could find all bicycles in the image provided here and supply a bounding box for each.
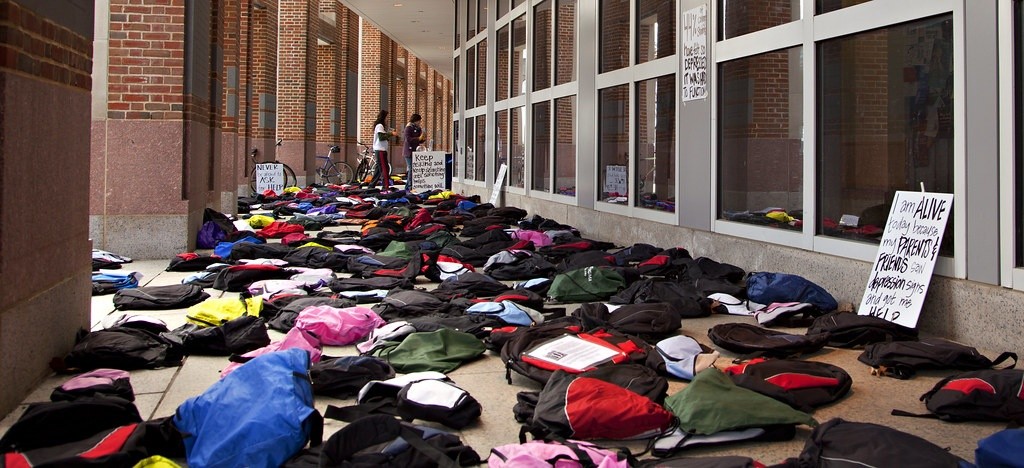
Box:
[251,149,287,195]
[275,140,297,187]
[357,141,392,185]
[316,144,355,186]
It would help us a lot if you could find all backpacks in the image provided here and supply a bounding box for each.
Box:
[0,171,1024,468]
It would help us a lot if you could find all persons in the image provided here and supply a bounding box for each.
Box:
[403,114,426,190]
[369,110,399,191]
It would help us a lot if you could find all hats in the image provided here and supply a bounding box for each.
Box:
[410,114,422,122]
